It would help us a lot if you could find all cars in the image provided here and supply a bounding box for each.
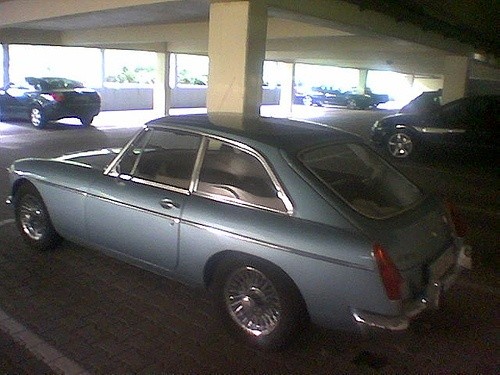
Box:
[370,96,500,163]
[5,112,474,352]
[0,76,101,130]
[399,91,443,111]
[293,84,390,110]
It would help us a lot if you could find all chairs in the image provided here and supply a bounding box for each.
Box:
[212,144,247,191]
[156,153,209,191]
